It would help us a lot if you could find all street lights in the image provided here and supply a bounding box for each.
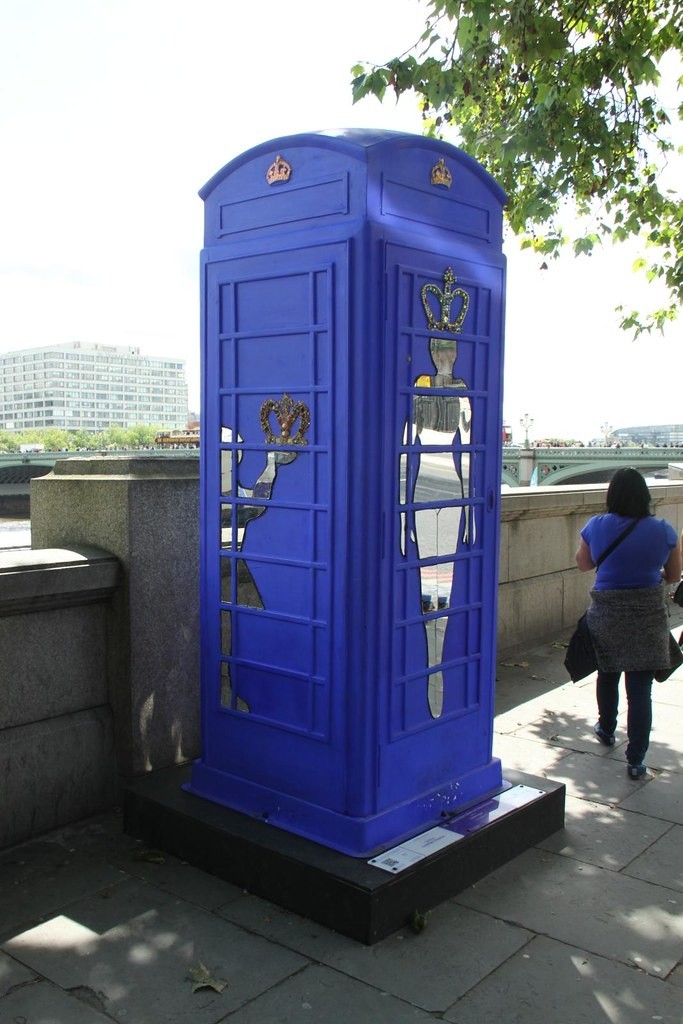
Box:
[520,413,535,447]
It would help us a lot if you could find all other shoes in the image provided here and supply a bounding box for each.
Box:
[595,722,617,746]
[627,762,647,780]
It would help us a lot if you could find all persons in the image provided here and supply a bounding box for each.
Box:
[576,468,683,779]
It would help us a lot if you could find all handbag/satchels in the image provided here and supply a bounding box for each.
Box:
[669,579,683,608]
